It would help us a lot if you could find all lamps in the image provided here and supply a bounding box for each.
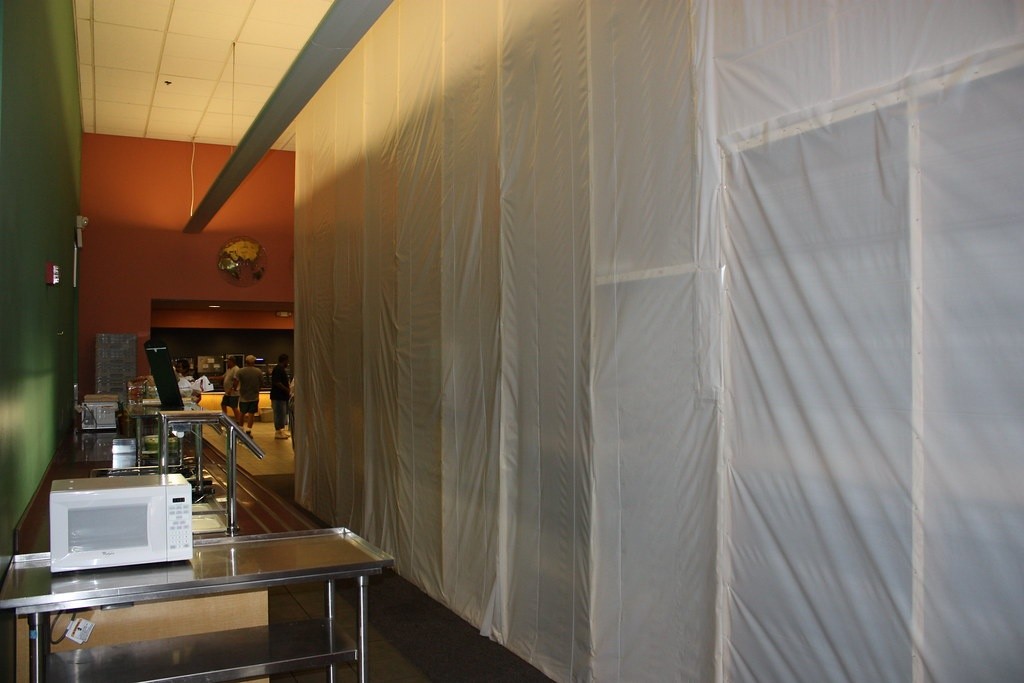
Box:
[182,0,391,234]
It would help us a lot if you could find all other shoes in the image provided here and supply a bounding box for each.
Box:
[246,431,253,439]
[275,432,290,439]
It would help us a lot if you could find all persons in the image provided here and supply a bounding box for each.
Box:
[209,356,241,426]
[270,354,291,439]
[175,358,190,376]
[237,355,264,439]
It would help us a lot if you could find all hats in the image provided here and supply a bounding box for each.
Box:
[246,355,256,363]
[224,355,236,362]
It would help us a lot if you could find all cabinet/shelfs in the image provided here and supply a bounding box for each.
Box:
[0,526,394,683]
